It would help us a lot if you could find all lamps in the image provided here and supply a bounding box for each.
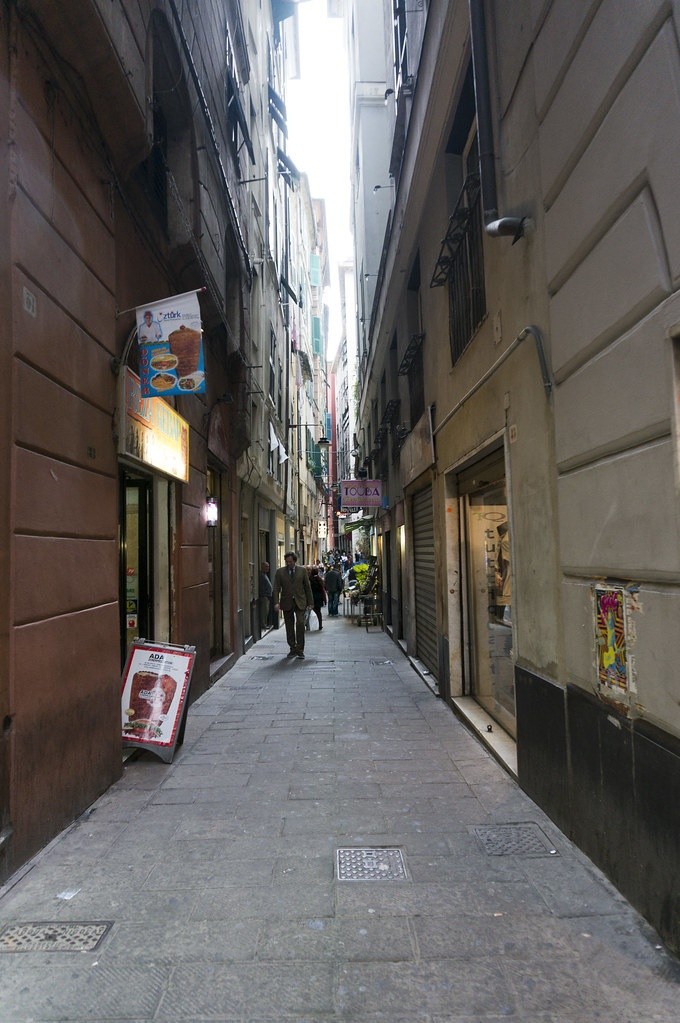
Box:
[206,495,219,527]
[287,423,332,448]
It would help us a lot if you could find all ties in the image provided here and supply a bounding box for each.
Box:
[290,568,293,576]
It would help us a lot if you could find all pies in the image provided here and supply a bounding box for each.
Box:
[178,371,205,391]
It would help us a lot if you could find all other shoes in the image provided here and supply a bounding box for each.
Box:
[287,653,298,658]
[297,653,305,659]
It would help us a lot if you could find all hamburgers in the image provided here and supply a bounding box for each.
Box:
[149,354,178,372]
[122,719,163,739]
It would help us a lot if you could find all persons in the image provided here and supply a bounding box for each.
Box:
[259,562,273,629]
[307,548,363,616]
[495,524,512,628]
[309,569,327,630]
[273,552,314,659]
[139,311,163,344]
